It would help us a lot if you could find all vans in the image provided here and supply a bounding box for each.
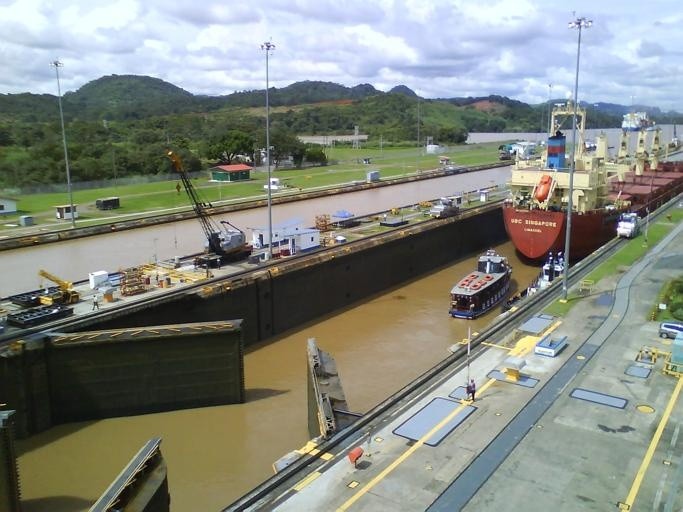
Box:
[658,321,683,339]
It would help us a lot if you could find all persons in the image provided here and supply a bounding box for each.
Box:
[216,258,220,270]
[470,379,475,401]
[91,295,99,310]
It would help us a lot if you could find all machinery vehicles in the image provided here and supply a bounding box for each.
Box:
[616,212,642,239]
[166,151,253,268]
[37,269,73,306]
[429,199,459,219]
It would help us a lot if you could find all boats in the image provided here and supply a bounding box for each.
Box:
[621,111,660,132]
[448,246,570,320]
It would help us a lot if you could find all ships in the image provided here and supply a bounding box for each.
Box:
[502,89,683,266]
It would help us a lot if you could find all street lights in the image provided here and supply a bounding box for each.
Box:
[49,61,76,227]
[258,41,277,257]
[559,16,593,304]
